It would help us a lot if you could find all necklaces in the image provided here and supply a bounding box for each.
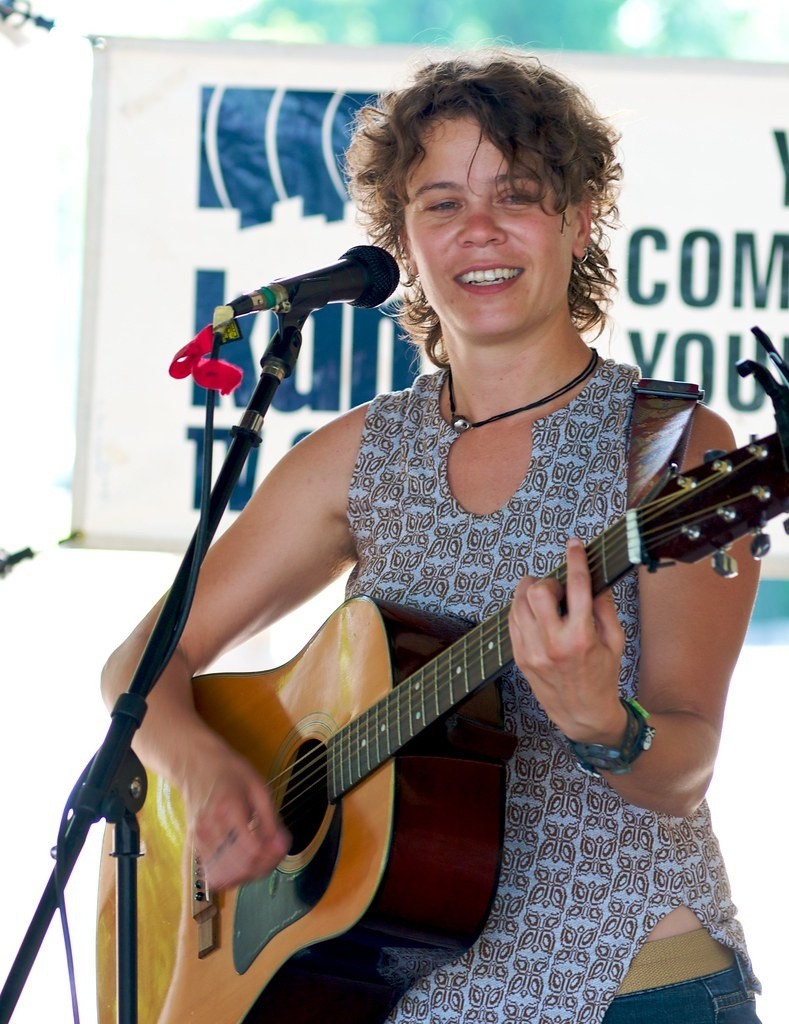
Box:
[447,347,598,430]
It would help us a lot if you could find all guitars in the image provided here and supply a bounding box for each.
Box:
[92,327,789,1024]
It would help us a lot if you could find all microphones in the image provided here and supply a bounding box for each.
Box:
[227,245,399,318]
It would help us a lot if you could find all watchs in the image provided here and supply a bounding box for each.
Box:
[567,696,654,785]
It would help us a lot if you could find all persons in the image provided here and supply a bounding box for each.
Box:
[98,55,766,1024]
